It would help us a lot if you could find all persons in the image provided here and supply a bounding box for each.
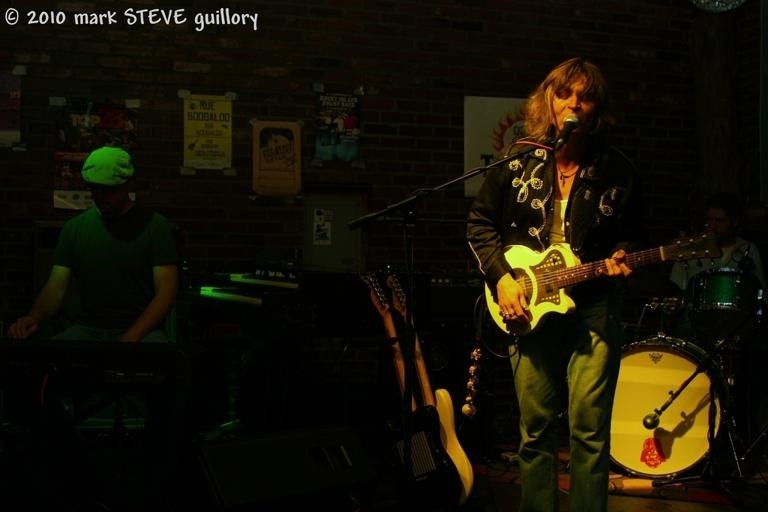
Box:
[2,143,179,349]
[464,55,651,512]
[665,195,766,301]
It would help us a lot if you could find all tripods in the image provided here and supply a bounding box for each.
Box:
[653,336,751,512]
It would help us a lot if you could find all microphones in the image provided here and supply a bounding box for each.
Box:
[644,398,667,427]
[554,115,578,145]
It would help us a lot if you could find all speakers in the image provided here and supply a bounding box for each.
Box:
[195,440,351,510]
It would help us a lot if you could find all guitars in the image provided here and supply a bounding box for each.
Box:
[485,235,722,335]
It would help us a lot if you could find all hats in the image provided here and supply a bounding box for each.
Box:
[80,145,135,186]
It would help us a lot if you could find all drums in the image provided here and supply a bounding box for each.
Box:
[687,267,743,316]
[608,338,732,477]
